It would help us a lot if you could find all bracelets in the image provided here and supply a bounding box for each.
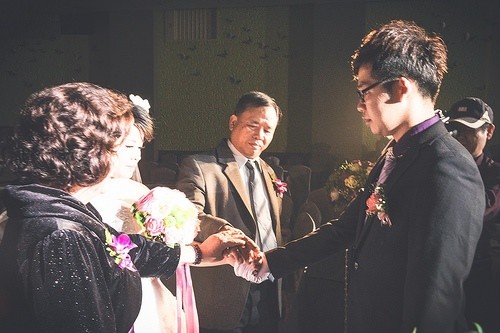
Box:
[196,243,204,265]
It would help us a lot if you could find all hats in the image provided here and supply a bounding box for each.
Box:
[444,96,494,130]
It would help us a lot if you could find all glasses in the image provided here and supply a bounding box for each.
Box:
[357,75,408,105]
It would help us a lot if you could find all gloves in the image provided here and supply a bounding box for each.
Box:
[233,250,270,284]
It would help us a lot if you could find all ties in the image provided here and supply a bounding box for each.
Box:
[245,159,275,283]
[364,147,394,223]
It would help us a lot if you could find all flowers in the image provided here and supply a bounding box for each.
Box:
[328,160,377,202]
[271,178,288,199]
[103,228,139,272]
[366,185,392,228]
[128,94,151,113]
[132,185,202,249]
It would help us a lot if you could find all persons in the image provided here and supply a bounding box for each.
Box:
[175,91,286,333]
[0,83,245,333]
[71,105,256,333]
[448,97,500,333]
[234,22,485,333]
